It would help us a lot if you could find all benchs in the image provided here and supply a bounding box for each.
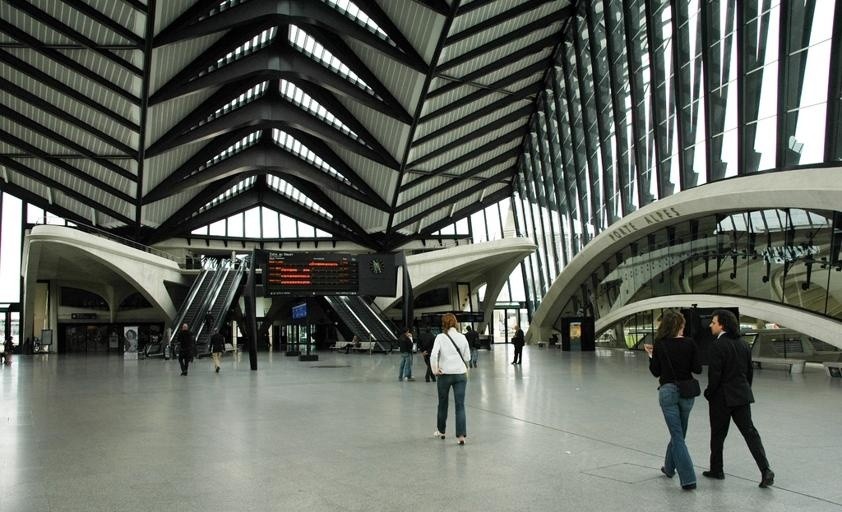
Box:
[206,342,240,358]
[822,359,841,382]
[330,340,377,356]
[748,352,805,375]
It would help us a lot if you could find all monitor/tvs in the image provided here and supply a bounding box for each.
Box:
[292,303,307,319]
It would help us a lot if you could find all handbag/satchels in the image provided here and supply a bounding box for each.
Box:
[679,378,700,399]
[723,375,755,407]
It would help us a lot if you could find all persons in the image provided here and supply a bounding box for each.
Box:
[510,324,526,366]
[207,327,226,374]
[429,312,473,446]
[397,327,417,382]
[4,335,15,366]
[643,308,703,491]
[176,323,194,377]
[702,308,776,490]
[419,326,437,382]
[338,335,360,354]
[406,328,416,380]
[462,325,480,369]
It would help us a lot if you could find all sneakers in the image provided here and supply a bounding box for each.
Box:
[661,466,674,478]
[469,361,479,368]
[181,371,187,376]
[457,439,465,445]
[703,470,724,480]
[434,431,446,440]
[425,376,436,382]
[216,366,220,373]
[681,483,696,491]
[398,376,417,381]
[759,469,775,488]
[511,361,521,364]
